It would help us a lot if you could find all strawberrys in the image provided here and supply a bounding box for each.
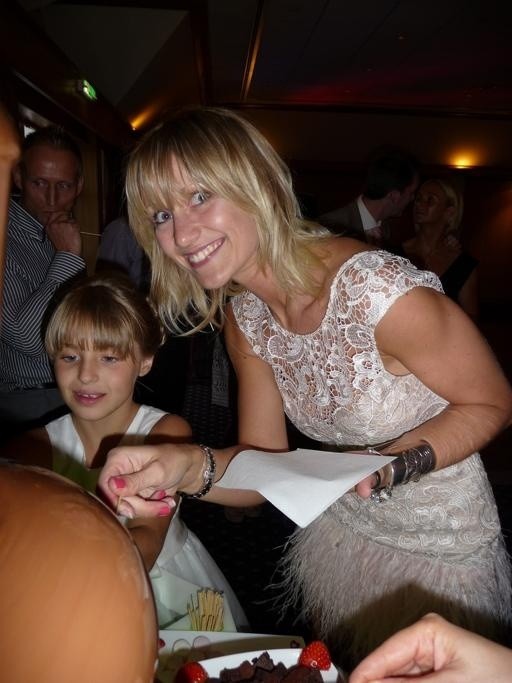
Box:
[300,640,330,670]
[176,662,208,683]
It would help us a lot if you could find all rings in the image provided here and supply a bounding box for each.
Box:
[373,471,381,489]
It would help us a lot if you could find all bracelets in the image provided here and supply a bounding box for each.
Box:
[176,444,216,499]
[372,440,437,489]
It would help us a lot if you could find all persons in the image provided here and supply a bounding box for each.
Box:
[1,127,87,437]
[97,107,512,654]
[32,278,248,633]
[319,145,511,336]
[350,612,512,683]
[95,215,145,285]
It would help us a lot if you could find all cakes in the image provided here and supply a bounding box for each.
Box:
[0,458,159,683]
